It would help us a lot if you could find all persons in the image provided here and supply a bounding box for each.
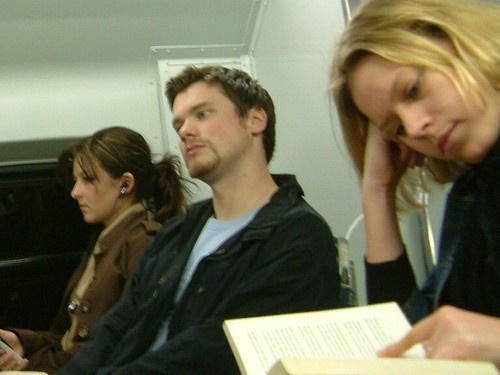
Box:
[54,64,342,375]
[328,0,500,360]
[0,127,202,375]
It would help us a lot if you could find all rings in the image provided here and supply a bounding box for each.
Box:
[424,345,434,352]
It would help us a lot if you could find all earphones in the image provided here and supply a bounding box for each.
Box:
[120,182,128,195]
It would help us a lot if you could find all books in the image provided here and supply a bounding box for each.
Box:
[222,299,500,375]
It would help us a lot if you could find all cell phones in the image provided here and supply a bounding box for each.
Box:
[0,336,23,365]
